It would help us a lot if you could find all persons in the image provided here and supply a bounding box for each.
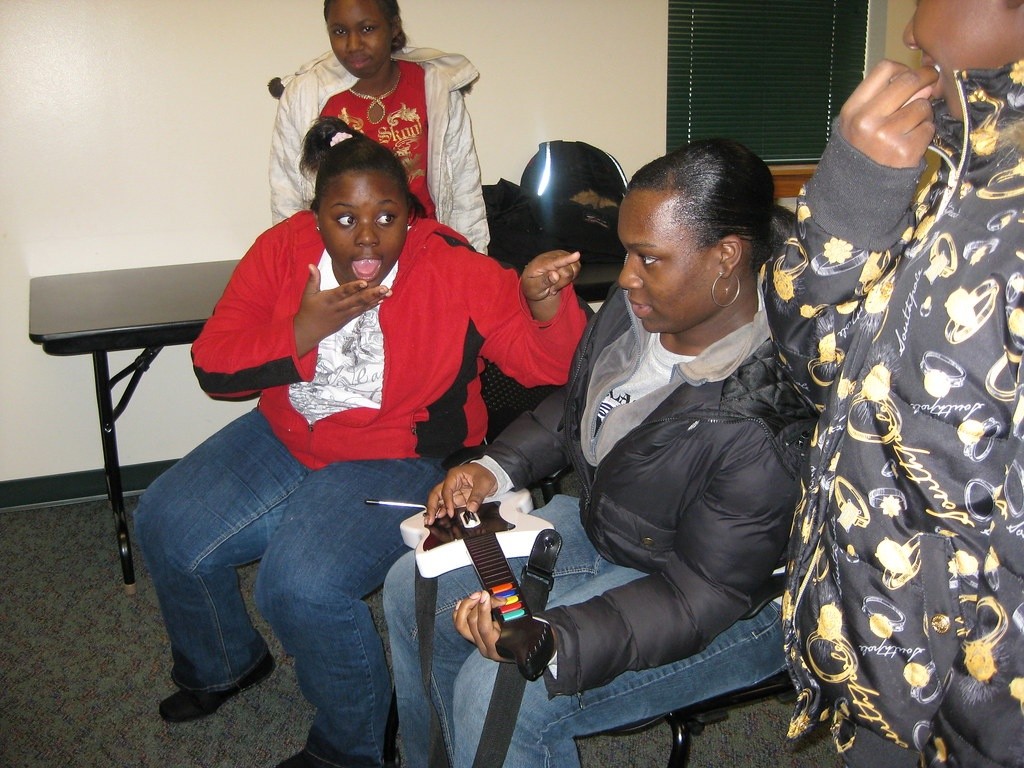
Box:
[269,1,492,449]
[132,116,593,768]
[759,1,1024,768]
[382,140,819,768]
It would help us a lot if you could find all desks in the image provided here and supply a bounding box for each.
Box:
[29,260,626,598]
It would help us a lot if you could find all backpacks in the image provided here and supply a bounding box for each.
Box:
[489,137,630,264]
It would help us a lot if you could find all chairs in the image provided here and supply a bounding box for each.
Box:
[480,290,600,501]
[385,673,794,767]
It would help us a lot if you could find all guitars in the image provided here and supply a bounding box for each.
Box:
[399,488,557,682]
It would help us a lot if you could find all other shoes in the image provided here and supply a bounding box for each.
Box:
[159,646,276,722]
[276,739,400,768]
